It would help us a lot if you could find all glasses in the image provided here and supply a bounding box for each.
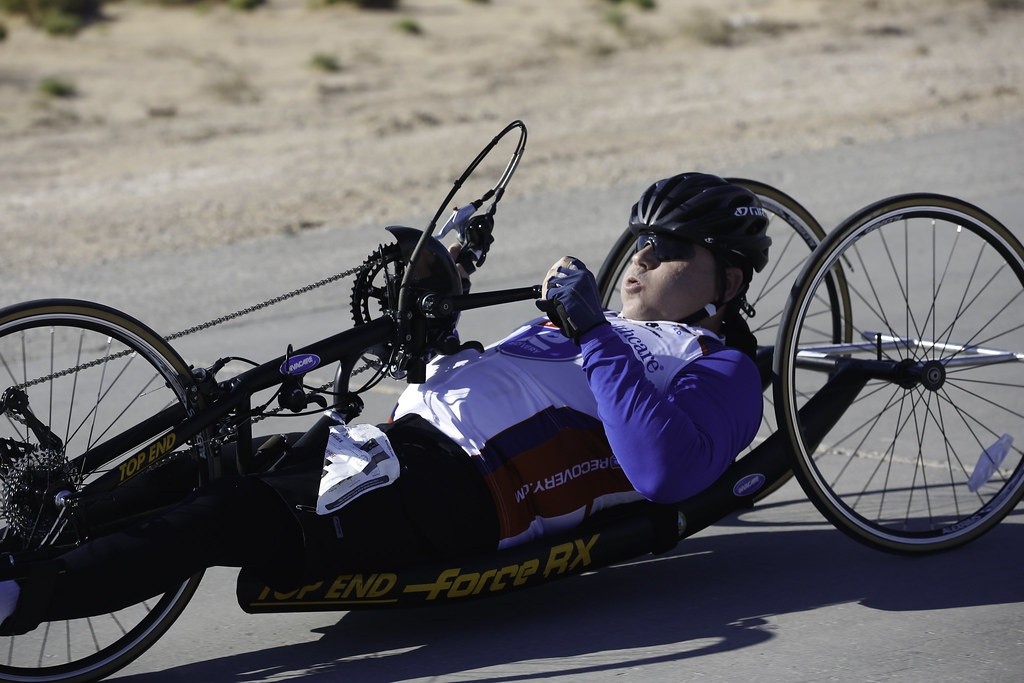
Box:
[636,233,696,261]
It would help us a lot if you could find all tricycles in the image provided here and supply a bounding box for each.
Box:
[1,117,1023,683]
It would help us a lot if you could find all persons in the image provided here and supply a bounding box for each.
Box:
[0,172,773,638]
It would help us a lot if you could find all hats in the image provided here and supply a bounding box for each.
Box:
[629,172,772,293]
